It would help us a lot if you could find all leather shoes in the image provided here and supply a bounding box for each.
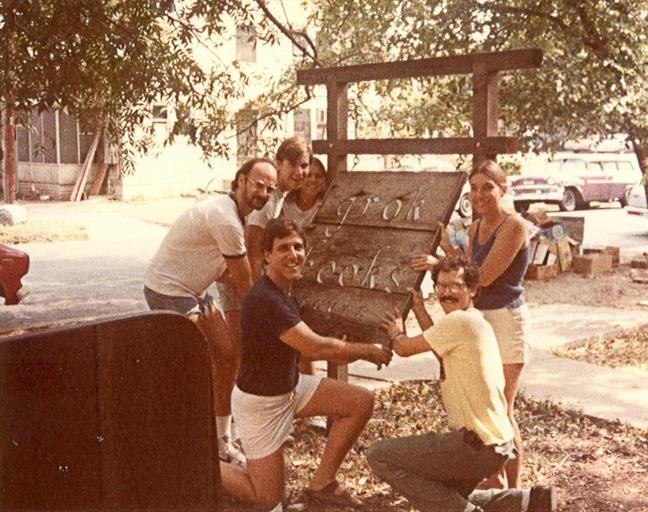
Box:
[527,485,557,512]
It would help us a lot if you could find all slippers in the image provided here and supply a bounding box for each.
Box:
[306,480,362,507]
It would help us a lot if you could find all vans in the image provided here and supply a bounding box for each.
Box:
[510,153,643,211]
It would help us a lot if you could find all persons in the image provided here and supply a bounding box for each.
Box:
[216,137,311,346]
[365,253,558,511]
[281,155,327,228]
[142,158,278,470]
[218,216,393,511]
[411,160,531,490]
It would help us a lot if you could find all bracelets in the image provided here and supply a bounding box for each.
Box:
[391,331,406,341]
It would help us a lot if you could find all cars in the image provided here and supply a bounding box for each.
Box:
[619,173,648,214]
[0,243,30,307]
[374,159,473,221]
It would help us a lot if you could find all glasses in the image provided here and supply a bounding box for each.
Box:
[433,282,464,292]
[249,177,276,193]
